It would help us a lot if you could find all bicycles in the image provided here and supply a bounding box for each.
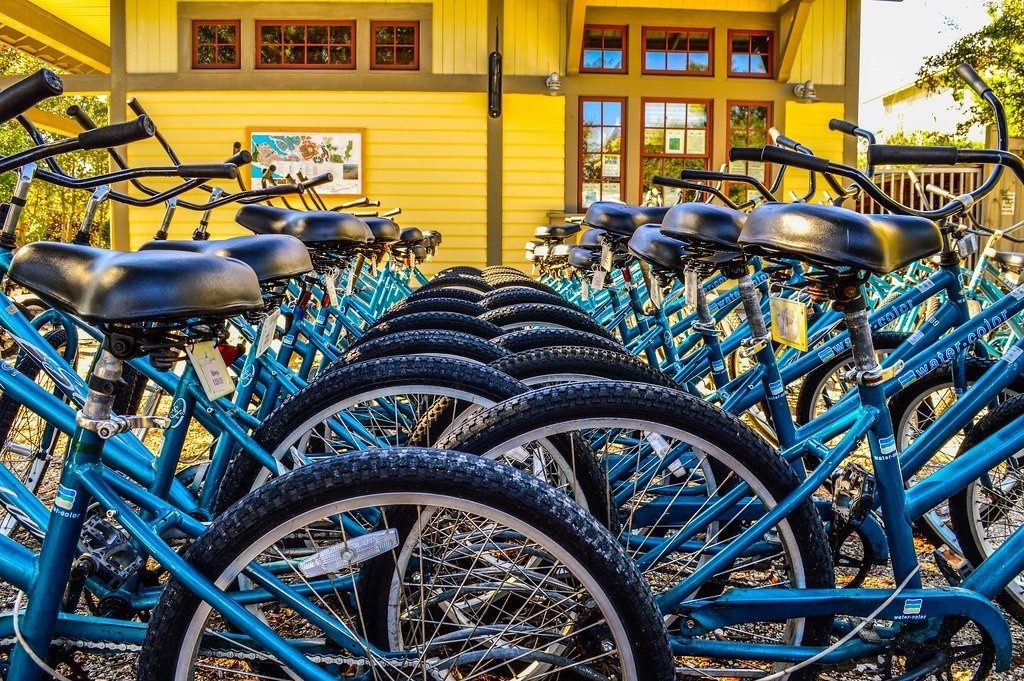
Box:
[0,55,1024,681]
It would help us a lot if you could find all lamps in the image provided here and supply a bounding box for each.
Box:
[793,79,817,104]
[546,72,562,97]
